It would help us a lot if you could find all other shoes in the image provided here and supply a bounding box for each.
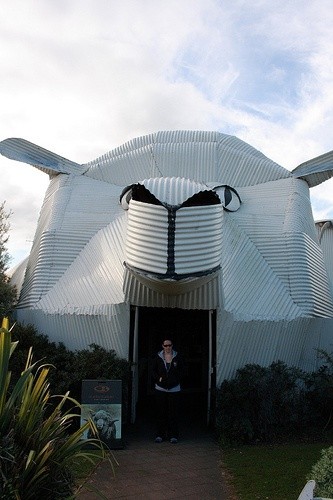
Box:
[170,437,179,443]
[155,436,163,443]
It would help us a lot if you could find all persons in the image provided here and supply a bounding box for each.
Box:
[151,338,187,444]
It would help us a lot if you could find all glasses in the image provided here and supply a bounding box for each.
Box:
[163,344,172,347]
